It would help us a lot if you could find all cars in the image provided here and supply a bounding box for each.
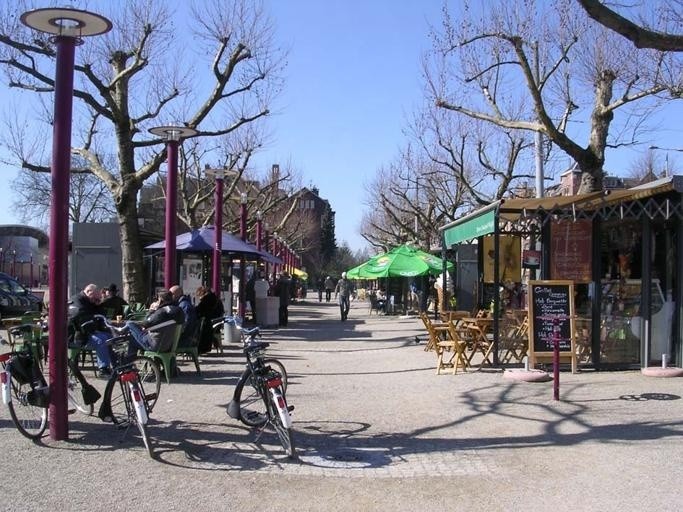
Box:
[1,273,42,318]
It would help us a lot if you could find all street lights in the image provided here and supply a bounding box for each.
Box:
[253,209,301,298]
[229,191,254,318]
[0,247,34,288]
[20,3,115,447]
[207,164,239,297]
[148,121,200,288]
[647,144,682,221]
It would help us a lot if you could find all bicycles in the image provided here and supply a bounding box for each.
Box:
[80,312,163,459]
[0,299,95,440]
[212,310,299,457]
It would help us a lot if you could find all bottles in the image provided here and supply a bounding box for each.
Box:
[665,289,672,302]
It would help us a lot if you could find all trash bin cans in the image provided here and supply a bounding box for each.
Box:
[224,316,242,342]
[255,296,280,329]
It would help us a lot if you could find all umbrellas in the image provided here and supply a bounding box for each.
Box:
[143,223,264,256]
[344,243,454,279]
[243,239,284,265]
[271,263,308,280]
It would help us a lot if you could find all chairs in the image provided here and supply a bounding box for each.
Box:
[20,299,226,385]
[420,301,613,378]
[368,290,407,317]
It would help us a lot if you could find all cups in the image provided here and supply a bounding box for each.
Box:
[116,315,122,322]
[618,304,624,311]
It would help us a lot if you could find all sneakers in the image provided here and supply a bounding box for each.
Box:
[96,368,113,380]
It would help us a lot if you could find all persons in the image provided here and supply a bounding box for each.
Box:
[410,276,430,313]
[334,271,354,320]
[375,288,386,311]
[316,276,323,302]
[245,268,307,328]
[322,275,334,302]
[64,283,224,372]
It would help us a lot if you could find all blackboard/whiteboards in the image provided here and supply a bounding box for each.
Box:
[528,280,575,356]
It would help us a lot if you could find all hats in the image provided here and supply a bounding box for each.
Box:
[341,271,347,277]
[103,282,121,292]
